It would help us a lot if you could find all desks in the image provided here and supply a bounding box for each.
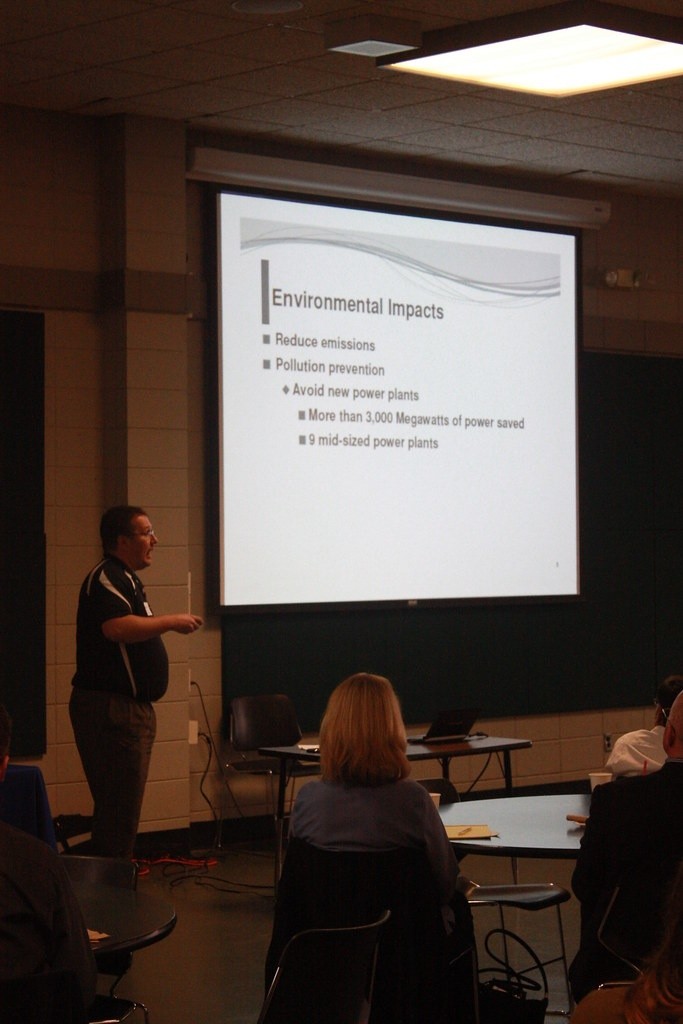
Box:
[434,794,591,858]
[53,874,177,954]
[257,734,531,886]
[0,766,54,853]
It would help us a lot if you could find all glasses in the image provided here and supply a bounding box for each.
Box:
[662,708,676,733]
[115,528,154,541]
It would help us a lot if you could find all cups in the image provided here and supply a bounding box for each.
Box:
[589,773,612,791]
[428,792,440,810]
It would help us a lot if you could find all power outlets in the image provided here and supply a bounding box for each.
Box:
[189,720,198,745]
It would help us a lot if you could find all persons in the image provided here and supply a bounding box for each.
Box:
[262,671,483,1024]
[570,687,683,1003]
[66,504,204,858]
[604,674,683,781]
[567,859,683,1024]
[0,702,98,1024]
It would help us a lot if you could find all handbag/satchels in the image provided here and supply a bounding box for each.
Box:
[478,929,549,1024]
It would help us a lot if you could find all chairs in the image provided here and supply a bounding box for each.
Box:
[58,853,148,1024]
[257,777,572,1024]
[211,693,300,861]
[598,839,683,1005]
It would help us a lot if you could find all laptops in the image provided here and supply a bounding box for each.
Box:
[406,709,482,744]
